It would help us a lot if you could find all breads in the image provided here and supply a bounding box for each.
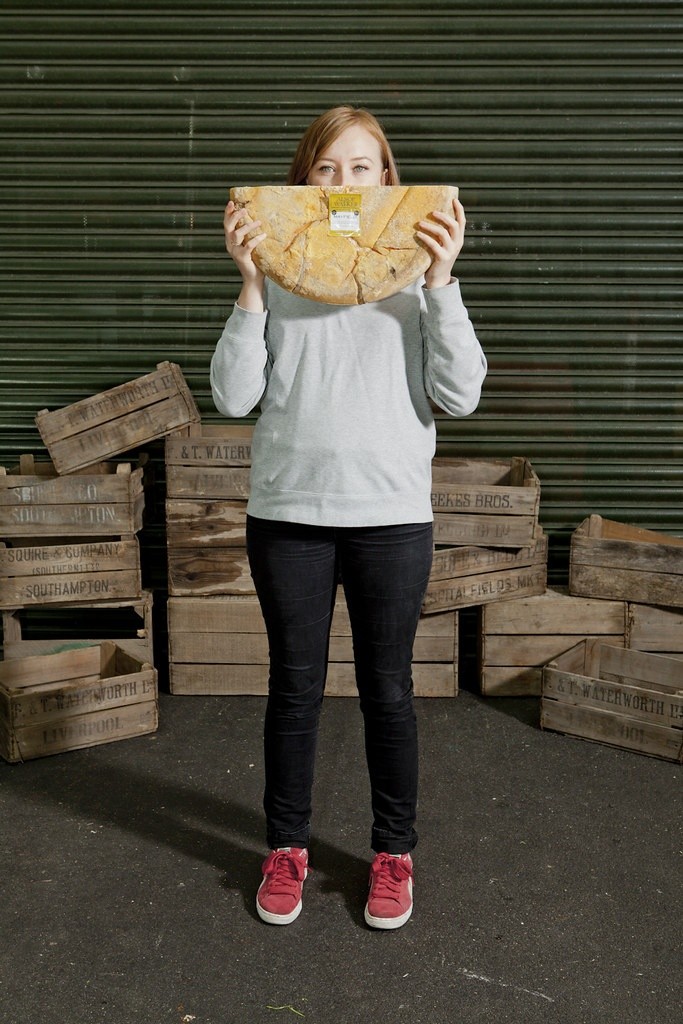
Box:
[230,185,459,305]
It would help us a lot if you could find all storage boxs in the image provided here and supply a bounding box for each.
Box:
[567,514,682,611]
[541,638,682,766]
[0,641,160,763]
[431,454,544,548]
[479,585,683,699]
[418,532,549,614]
[33,360,201,477]
[0,421,459,700]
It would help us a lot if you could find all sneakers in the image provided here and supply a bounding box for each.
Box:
[364,852,416,929]
[255,847,309,925]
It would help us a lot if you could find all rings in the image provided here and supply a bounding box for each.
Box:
[230,241,242,246]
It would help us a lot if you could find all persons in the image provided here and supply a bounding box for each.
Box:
[204,106,490,932]
